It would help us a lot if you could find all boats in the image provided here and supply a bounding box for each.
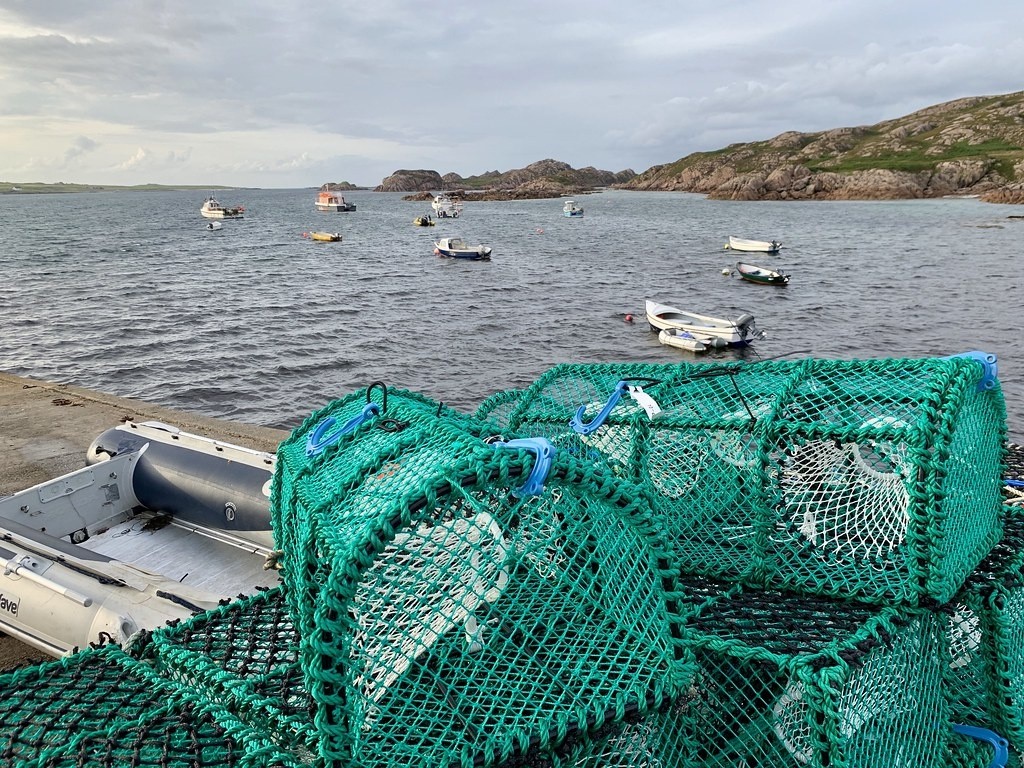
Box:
[0,421,276,658]
[659,328,726,353]
[310,231,342,241]
[315,192,357,211]
[729,235,782,252]
[736,261,791,285]
[414,214,434,227]
[563,201,584,218]
[435,202,459,218]
[645,299,767,348]
[432,196,463,212]
[205,221,222,231]
[200,199,245,219]
[434,238,491,259]
